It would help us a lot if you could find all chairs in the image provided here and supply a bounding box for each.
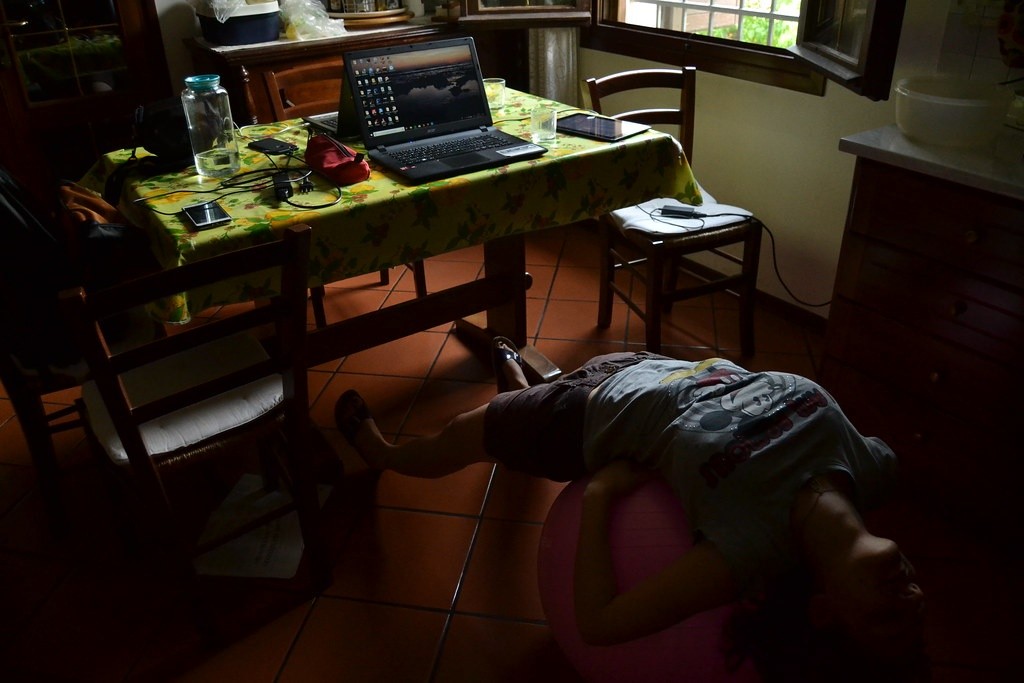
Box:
[586,65,762,361]
[1,346,135,545]
[58,223,334,640]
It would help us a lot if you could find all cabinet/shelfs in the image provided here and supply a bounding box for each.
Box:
[181,12,528,129]
[814,123,1024,554]
[0,0,183,255]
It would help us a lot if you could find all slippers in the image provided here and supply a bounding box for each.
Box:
[491,336,524,396]
[334,390,389,478]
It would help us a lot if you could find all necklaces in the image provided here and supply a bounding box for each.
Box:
[796,477,840,537]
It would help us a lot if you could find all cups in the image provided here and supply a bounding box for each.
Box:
[482,78,506,109]
[530,106,557,142]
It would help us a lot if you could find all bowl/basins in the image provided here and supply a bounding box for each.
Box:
[894,77,1016,149]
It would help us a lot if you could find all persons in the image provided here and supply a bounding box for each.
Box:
[334,335,932,683]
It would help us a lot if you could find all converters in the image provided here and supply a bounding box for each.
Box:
[273,171,293,200]
[661,206,694,219]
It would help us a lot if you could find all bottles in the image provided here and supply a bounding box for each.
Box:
[181,74,240,178]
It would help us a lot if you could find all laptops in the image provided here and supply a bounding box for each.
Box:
[302,66,363,143]
[342,36,548,182]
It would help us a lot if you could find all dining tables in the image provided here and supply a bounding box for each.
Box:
[98,78,687,593]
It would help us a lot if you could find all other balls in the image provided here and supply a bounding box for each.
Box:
[533,451,782,683]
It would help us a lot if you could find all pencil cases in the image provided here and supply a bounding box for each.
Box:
[303,124,370,187]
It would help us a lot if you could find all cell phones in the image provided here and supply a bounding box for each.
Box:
[182,201,231,228]
[248,138,295,155]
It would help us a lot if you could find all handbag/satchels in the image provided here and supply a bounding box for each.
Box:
[103,95,221,206]
[305,132,369,186]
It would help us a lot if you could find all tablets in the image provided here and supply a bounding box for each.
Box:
[556,113,648,142]
